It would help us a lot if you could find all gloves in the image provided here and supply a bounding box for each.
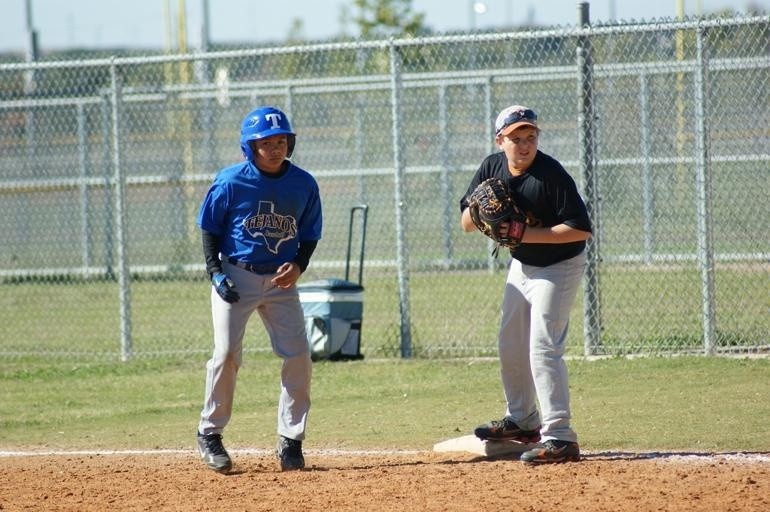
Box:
[211,271,240,303]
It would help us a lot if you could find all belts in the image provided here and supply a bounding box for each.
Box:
[219,252,255,273]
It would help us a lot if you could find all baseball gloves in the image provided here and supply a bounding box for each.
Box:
[466,177,530,249]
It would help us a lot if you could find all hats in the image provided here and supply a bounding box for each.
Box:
[495,105,541,137]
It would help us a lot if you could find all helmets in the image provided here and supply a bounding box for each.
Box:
[239,107,296,161]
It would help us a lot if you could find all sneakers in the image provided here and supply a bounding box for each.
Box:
[276,435,305,472]
[198,431,232,471]
[521,440,580,463]
[474,418,542,445]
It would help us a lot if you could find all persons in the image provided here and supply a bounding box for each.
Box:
[196,106,323,472]
[459,104,593,465]
